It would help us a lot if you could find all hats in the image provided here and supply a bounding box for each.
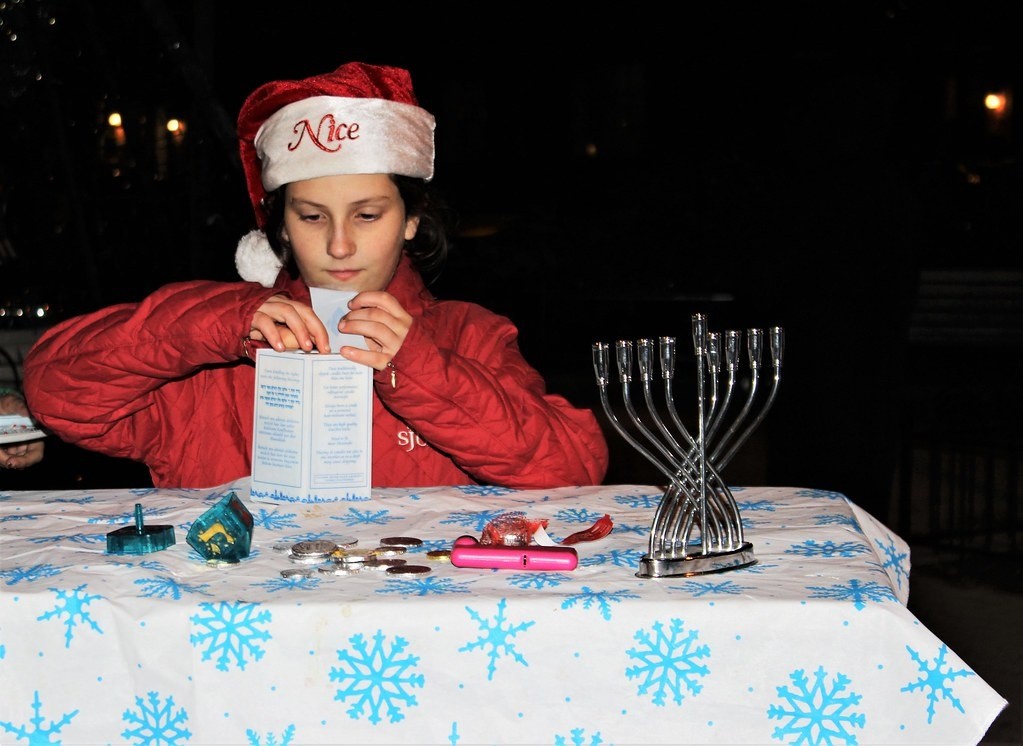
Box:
[235,63,436,291]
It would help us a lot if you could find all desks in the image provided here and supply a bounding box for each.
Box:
[0,484,1013,746]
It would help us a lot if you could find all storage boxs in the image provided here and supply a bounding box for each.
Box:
[249,347,374,502]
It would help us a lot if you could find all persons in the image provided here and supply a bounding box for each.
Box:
[0,59,610,489]
